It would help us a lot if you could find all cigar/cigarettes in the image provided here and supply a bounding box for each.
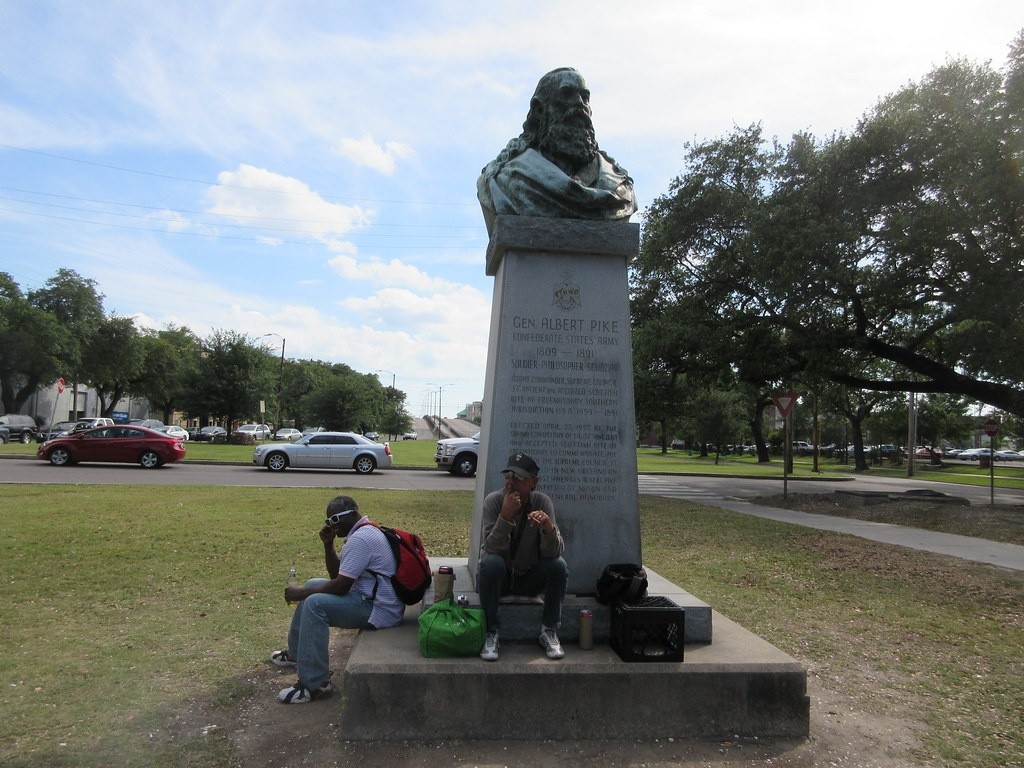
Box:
[527,515,541,524]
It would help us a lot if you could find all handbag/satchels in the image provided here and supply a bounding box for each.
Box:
[595,563,648,605]
[418,598,487,659]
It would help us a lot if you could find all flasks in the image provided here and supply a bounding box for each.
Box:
[579,610,593,650]
[431,566,456,603]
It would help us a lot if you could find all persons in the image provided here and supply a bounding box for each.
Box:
[475,452,569,660]
[271,496,405,703]
[476,68,638,237]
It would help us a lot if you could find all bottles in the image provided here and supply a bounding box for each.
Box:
[288,568,299,608]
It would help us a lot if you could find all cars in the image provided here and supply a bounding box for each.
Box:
[158,426,199,441]
[270,428,302,441]
[253,431,393,474]
[303,427,326,437]
[35,418,115,442]
[193,426,227,442]
[402,431,418,440]
[37,424,186,469]
[365,432,380,442]
[670,439,1024,462]
[0,428,10,444]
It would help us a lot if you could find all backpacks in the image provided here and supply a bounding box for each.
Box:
[353,522,432,606]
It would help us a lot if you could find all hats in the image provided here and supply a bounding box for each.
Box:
[500,453,539,476]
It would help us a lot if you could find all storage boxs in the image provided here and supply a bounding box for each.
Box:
[611,597,686,662]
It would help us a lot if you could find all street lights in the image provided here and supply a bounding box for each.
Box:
[378,369,395,398]
[423,382,455,438]
[265,333,285,433]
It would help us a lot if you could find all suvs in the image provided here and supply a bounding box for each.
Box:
[122,419,165,434]
[232,424,271,440]
[434,431,480,476]
[0,413,36,445]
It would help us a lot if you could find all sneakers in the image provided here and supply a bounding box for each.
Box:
[479,633,500,659]
[538,624,564,658]
[270,651,297,666]
[279,671,337,704]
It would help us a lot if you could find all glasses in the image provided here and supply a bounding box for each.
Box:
[325,510,354,527]
[503,471,527,482]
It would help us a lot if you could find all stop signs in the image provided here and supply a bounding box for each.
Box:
[57,377,66,394]
[985,420,998,437]
